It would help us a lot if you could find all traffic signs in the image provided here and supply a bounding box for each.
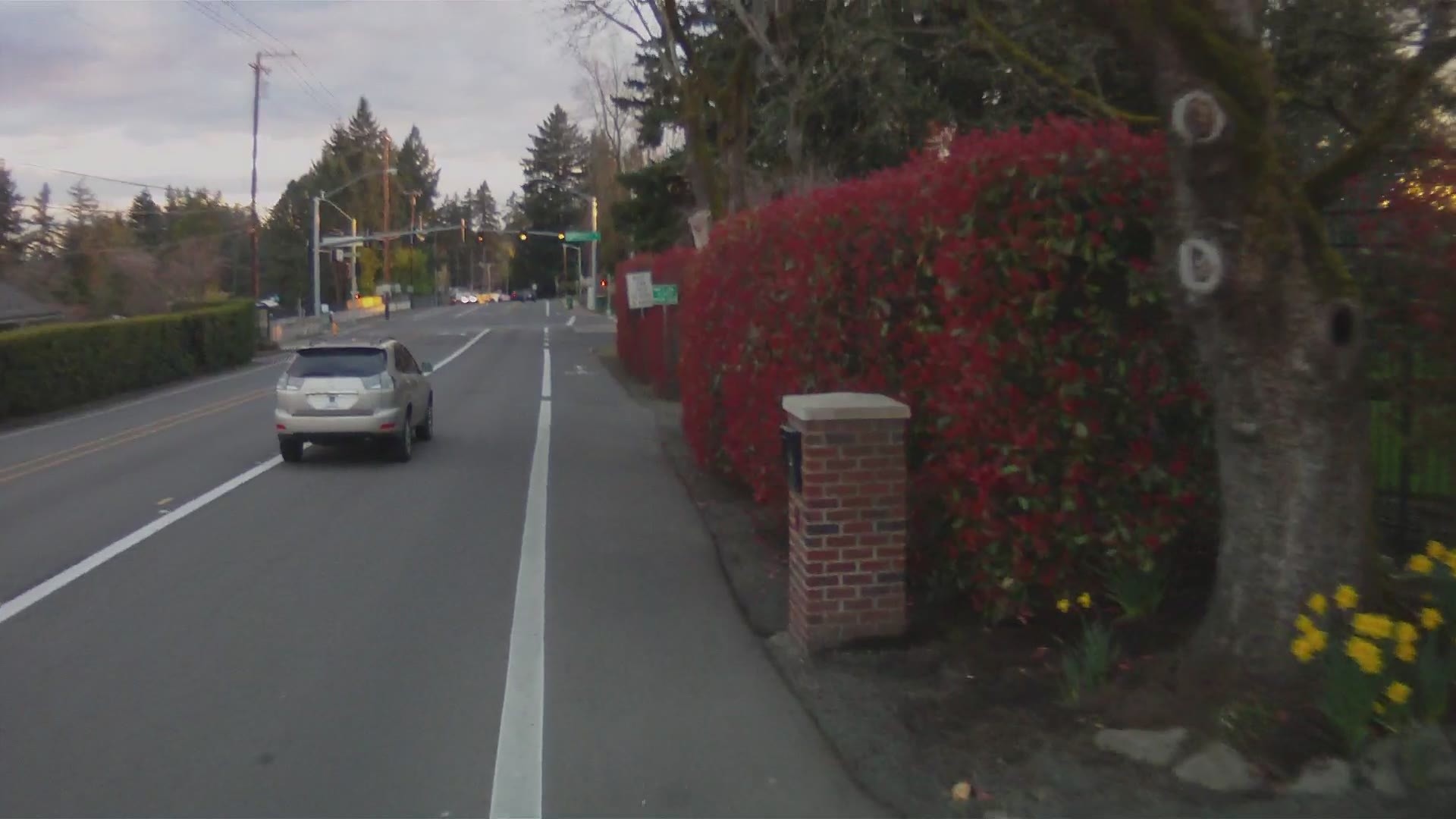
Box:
[563,231,601,243]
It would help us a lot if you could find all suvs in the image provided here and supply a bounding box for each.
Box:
[274,338,434,465]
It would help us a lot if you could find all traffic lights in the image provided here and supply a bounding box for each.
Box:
[519,230,528,246]
[476,230,484,248]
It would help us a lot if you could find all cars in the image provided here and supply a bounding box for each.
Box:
[448,285,538,306]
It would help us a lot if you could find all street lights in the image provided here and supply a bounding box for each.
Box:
[561,242,583,297]
[566,188,599,309]
[312,166,400,320]
